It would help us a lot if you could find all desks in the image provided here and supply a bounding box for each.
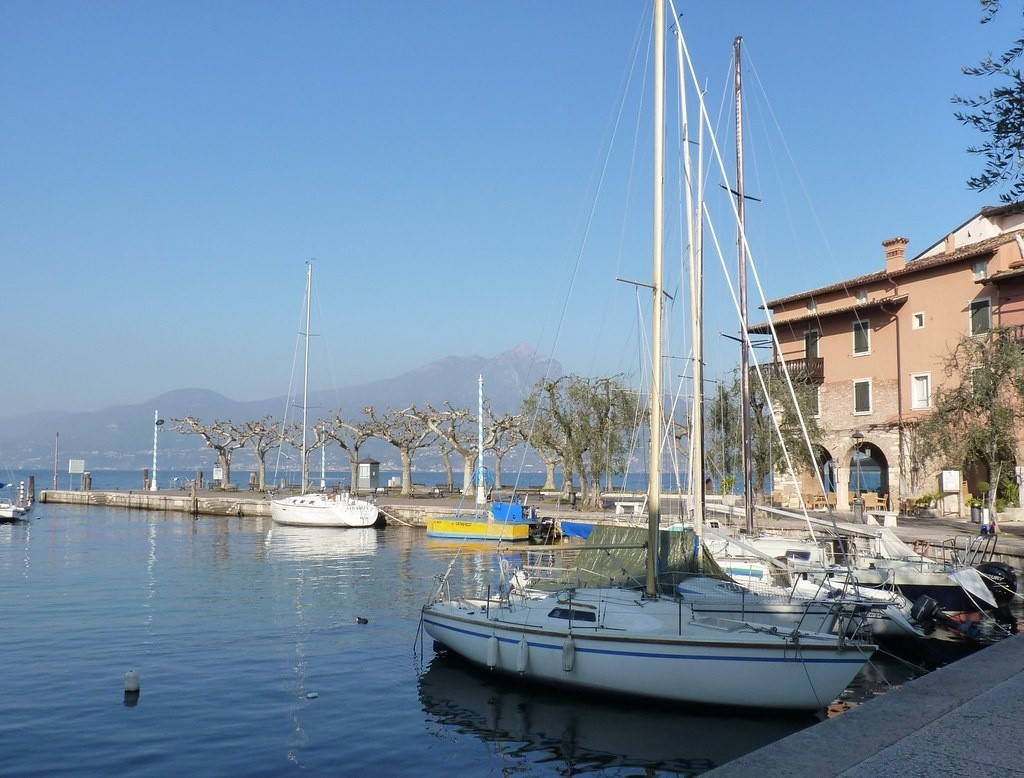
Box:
[859,497,886,501]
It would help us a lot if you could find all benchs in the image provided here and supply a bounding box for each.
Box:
[502,485,515,489]
[383,486,402,497]
[219,483,240,492]
[352,487,378,498]
[408,488,436,499]
[529,485,544,489]
[439,487,462,495]
[614,502,644,514]
[287,483,309,494]
[513,489,544,501]
[562,487,581,492]
[260,484,280,495]
[602,487,624,491]
[332,485,351,494]
[208,483,221,490]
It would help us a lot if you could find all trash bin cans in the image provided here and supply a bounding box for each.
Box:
[971,502,981,524]
[449,484,453,493]
[568,492,576,509]
[981,508,990,524]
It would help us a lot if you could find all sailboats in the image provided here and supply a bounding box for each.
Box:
[0,459,30,522]
[421,0,1023,709]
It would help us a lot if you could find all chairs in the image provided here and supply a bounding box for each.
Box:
[848,491,856,512]
[876,494,888,511]
[827,492,836,510]
[865,493,878,511]
[772,491,788,507]
[905,498,918,517]
[801,493,809,508]
[807,493,823,510]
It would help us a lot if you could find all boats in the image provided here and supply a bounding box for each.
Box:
[424,466,569,541]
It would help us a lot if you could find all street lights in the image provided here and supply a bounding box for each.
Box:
[149,409,160,491]
[269,258,379,527]
[318,421,327,491]
[477,373,487,505]
[851,429,866,525]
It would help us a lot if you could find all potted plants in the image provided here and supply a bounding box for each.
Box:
[720,475,735,506]
[976,481,989,524]
